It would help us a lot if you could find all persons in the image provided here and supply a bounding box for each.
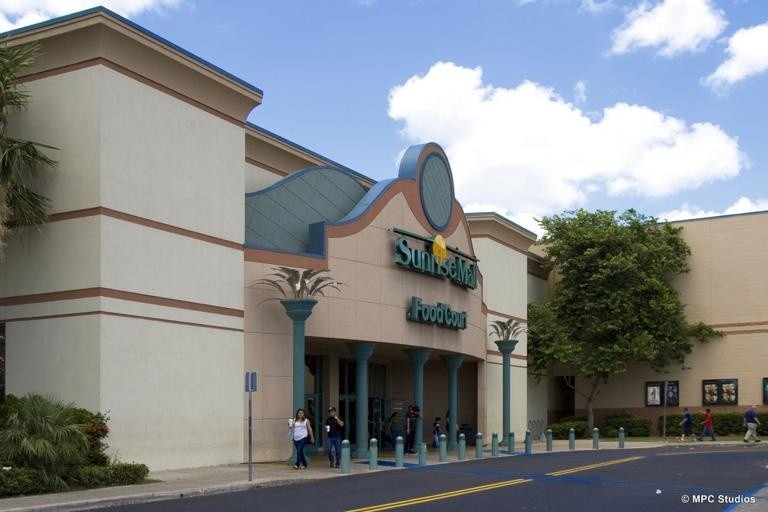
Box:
[288,409,315,469]
[695,408,717,442]
[325,407,345,468]
[742,405,762,443]
[677,407,697,443]
[666,385,678,405]
[432,416,445,449]
[376,405,423,455]
[445,410,450,438]
